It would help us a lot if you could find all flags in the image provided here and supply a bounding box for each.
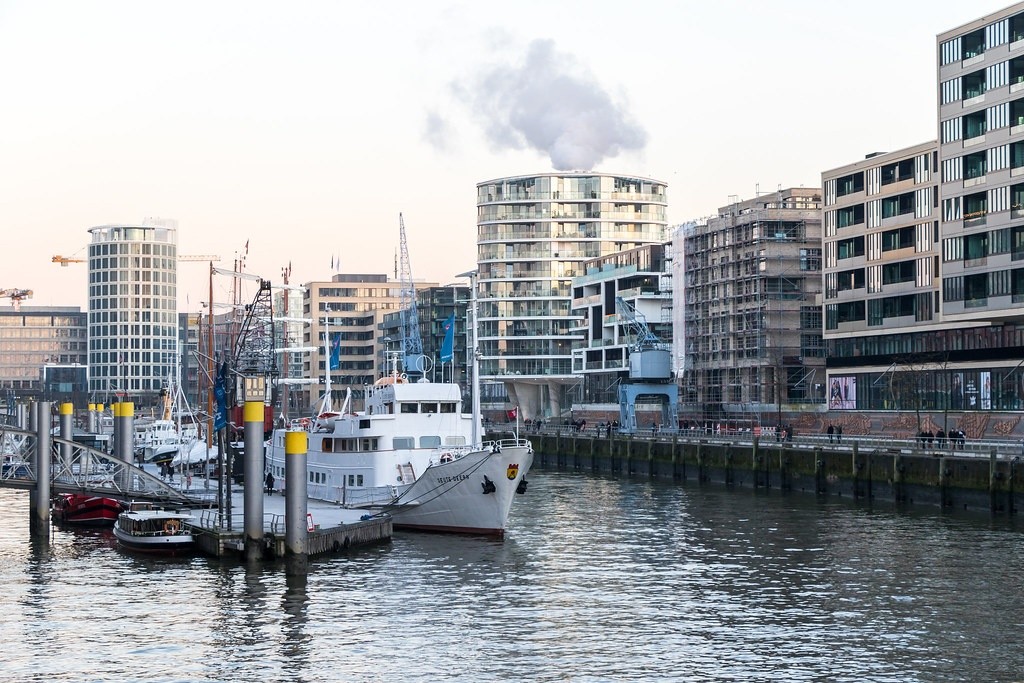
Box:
[330,336,340,370]
[506,407,517,420]
[439,311,454,363]
[212,361,226,434]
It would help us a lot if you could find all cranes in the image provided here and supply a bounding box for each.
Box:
[614,296,679,435]
[397,211,425,383]
[52,239,221,273]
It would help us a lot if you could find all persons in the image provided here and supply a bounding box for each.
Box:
[651,422,657,441]
[827,423,842,444]
[564,418,586,433]
[775,424,793,442]
[916,428,966,450]
[138,451,143,467]
[953,374,990,409]
[595,420,621,439]
[831,378,849,409]
[524,418,546,433]
[684,420,688,437]
[265,473,275,496]
[482,417,495,427]
[161,462,175,482]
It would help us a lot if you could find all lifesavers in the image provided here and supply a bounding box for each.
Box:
[163,519,179,535]
[301,418,310,427]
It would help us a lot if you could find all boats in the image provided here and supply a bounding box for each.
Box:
[373,376,409,386]
[112,499,195,552]
[255,352,537,540]
[97,348,221,476]
[50,490,124,527]
